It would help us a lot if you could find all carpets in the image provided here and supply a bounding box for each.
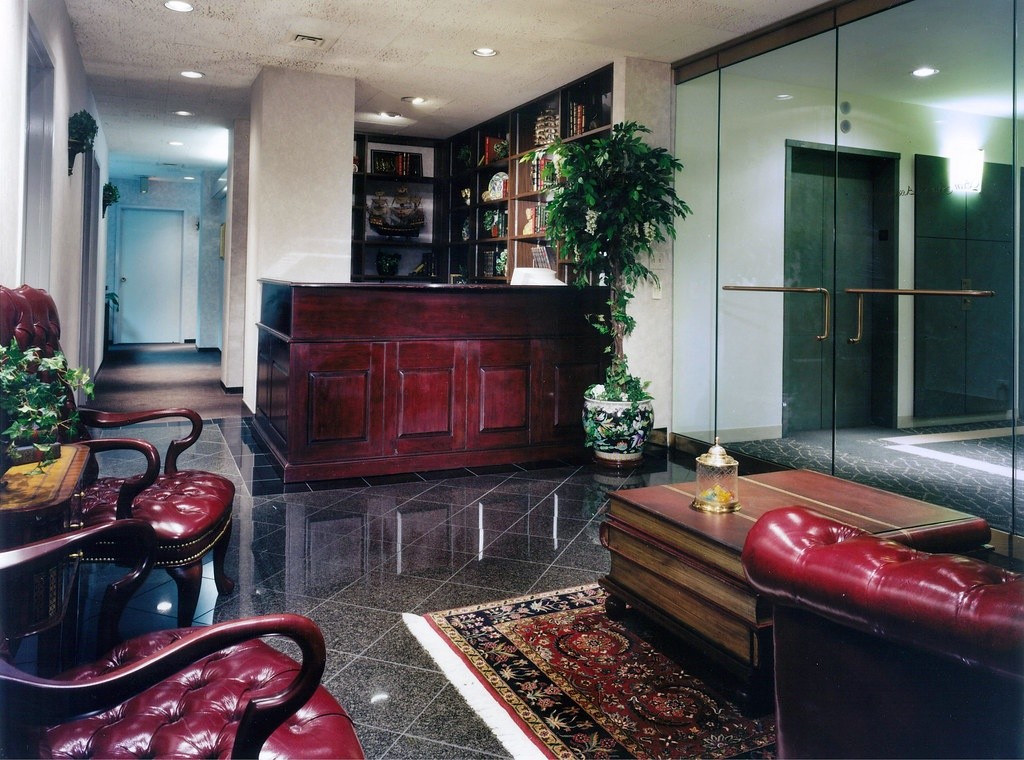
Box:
[402,583,778,760]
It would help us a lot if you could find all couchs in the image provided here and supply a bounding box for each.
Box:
[741,505,1024,760]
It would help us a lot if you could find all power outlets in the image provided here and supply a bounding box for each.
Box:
[650,250,666,270]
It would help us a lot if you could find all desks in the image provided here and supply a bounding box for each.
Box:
[0,444,90,520]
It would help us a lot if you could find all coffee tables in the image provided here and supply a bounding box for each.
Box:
[596,468,995,715]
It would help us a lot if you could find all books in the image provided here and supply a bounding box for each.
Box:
[531,245,557,278]
[485,136,505,165]
[483,250,505,277]
[503,179,510,198]
[533,201,554,233]
[569,101,602,136]
[529,149,558,191]
[492,209,508,238]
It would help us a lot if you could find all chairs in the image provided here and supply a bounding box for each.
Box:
[0,284,234,630]
[0,519,363,760]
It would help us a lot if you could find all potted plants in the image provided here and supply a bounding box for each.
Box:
[68,108,100,175]
[0,336,96,492]
[516,119,694,469]
[103,181,120,218]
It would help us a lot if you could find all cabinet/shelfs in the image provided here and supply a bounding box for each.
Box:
[351,139,441,282]
[441,57,677,285]
